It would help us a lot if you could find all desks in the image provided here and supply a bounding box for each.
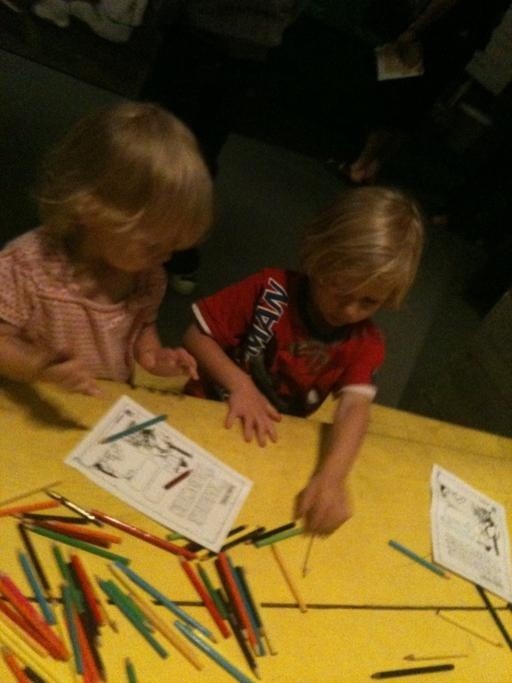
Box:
[1,364,511,683]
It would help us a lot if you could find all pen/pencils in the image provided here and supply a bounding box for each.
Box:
[0,470,316,683]
[101,416,167,445]
[372,664,454,679]
[389,539,450,580]
[477,584,512,650]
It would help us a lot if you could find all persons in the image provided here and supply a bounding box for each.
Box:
[177,180,428,537]
[1,97,217,401]
